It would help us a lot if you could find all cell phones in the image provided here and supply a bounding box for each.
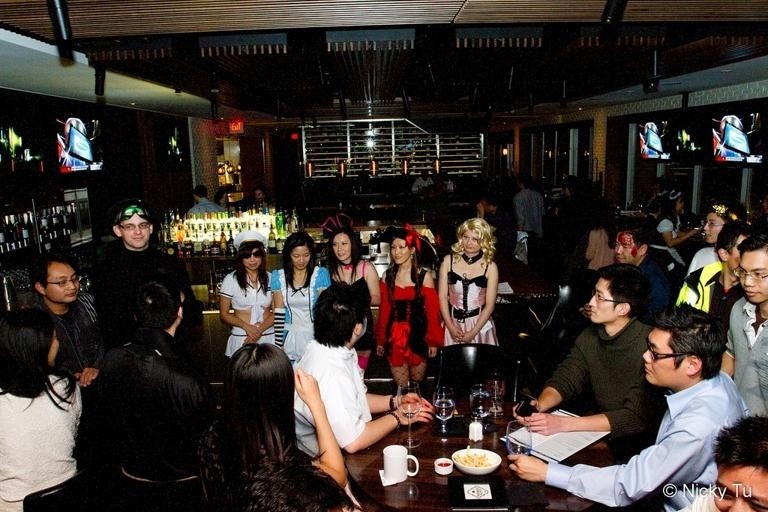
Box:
[516,397,540,419]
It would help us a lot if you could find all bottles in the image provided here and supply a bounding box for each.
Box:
[158,198,297,257]
[0,202,77,253]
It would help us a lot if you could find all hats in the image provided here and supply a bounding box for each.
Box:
[108,198,151,225]
[234,230,267,251]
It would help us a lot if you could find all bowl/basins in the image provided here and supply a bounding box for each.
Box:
[434,458,453,476]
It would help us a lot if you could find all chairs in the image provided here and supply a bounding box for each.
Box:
[20,466,94,512]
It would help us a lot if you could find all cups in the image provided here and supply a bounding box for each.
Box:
[382,482,419,509]
[506,420,532,456]
[432,387,455,436]
[383,445,419,482]
[396,381,422,449]
[469,384,493,431]
[487,379,507,417]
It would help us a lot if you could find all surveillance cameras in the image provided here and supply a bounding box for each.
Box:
[641,75,662,96]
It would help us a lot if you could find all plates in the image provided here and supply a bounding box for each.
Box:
[451,448,502,476]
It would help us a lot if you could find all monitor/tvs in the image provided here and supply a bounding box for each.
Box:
[46,113,123,180]
[635,108,768,169]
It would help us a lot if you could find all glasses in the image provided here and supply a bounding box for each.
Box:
[119,221,151,231]
[700,219,723,226]
[243,251,263,258]
[733,266,768,280]
[591,289,624,304]
[46,276,80,287]
[645,336,686,361]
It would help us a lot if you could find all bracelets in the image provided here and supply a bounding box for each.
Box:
[389,393,398,412]
[384,410,401,429]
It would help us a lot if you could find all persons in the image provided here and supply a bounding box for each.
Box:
[439,217,499,347]
[512,262,654,439]
[85,199,203,345]
[97,281,212,486]
[30,254,103,387]
[375,224,443,388]
[584,196,618,270]
[188,185,225,212]
[325,227,381,375]
[291,280,434,459]
[614,222,672,312]
[270,232,330,362]
[686,199,747,274]
[0,308,81,512]
[677,416,768,512]
[194,342,360,511]
[646,188,698,282]
[411,169,433,191]
[513,174,544,240]
[720,229,767,418]
[475,198,497,220]
[218,231,276,359]
[507,300,749,512]
[254,186,269,208]
[675,222,754,340]
